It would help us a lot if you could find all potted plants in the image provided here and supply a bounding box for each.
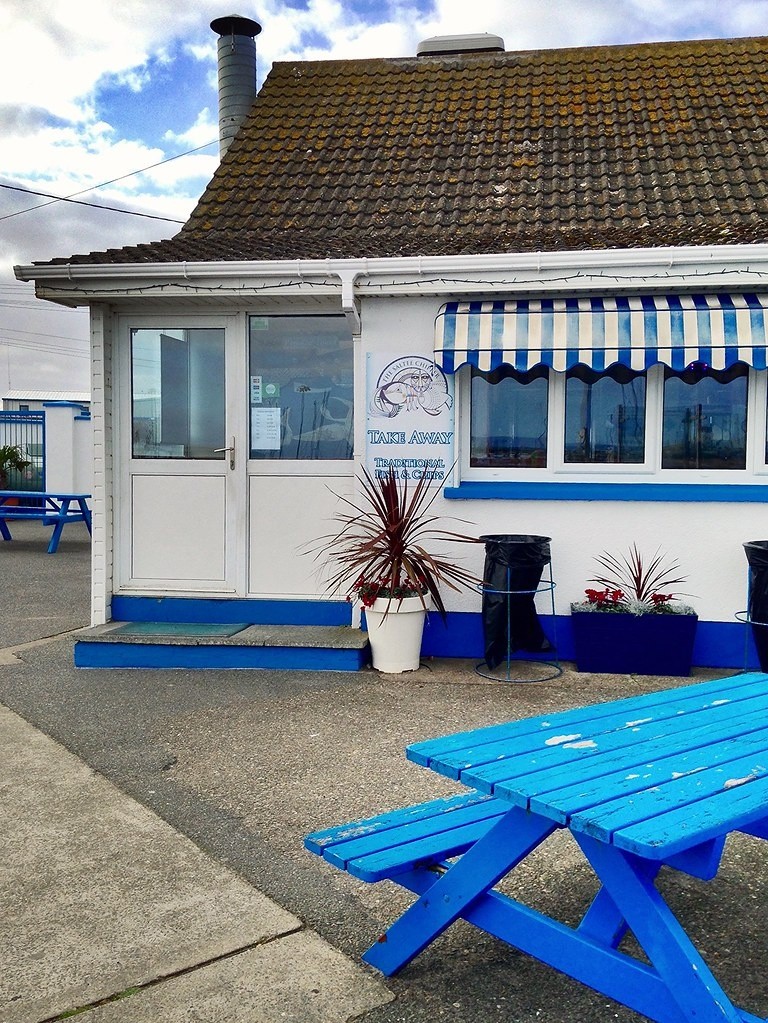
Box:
[313,459,484,676]
[570,546,701,675]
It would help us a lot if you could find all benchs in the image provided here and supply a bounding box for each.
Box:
[305,781,526,883]
[0,512,74,552]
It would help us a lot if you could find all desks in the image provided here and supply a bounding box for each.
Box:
[352,671,768,1022]
[0,507,93,540]
[0,489,93,553]
